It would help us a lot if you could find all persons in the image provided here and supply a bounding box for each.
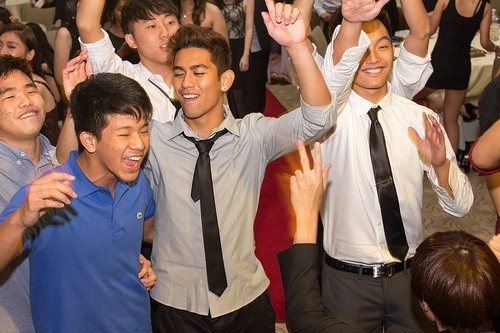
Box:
[0,72,156,333]
[276,140,500,333]
[0,0,500,236]
[308,0,474,333]
[0,54,156,333]
[55,0,335,333]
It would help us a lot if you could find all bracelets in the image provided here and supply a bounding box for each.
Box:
[493,45,496,51]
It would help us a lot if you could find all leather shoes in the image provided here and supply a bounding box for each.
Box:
[460,157,470,174]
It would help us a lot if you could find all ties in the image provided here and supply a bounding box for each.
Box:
[182,128,228,297]
[367,105,409,262]
[148,79,181,121]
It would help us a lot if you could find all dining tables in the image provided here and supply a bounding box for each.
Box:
[388,25,500,106]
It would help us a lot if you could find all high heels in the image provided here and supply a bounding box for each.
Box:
[456,150,464,170]
[463,103,479,122]
[270,72,291,85]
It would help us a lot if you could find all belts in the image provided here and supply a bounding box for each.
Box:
[324,253,413,278]
